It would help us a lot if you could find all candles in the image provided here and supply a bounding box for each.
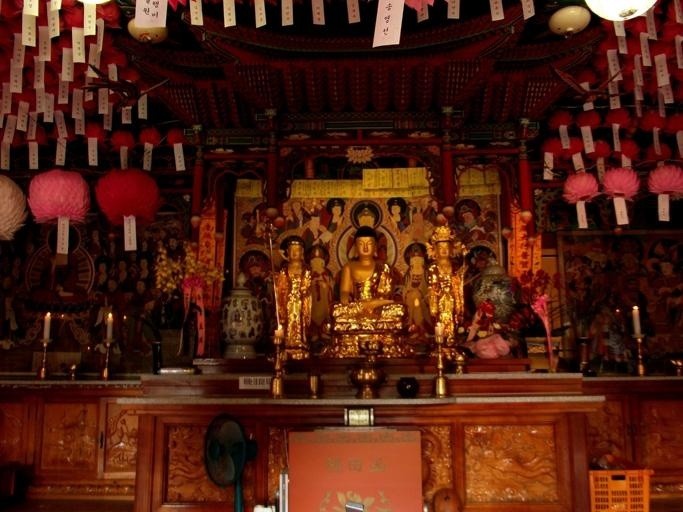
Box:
[43,312,51,340]
[106,312,113,341]
[632,305,640,335]
[274,324,285,336]
[434,321,442,336]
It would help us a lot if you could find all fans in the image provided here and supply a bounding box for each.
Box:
[203,411,259,511]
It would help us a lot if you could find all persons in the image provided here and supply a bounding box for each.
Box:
[239,189,501,373]
